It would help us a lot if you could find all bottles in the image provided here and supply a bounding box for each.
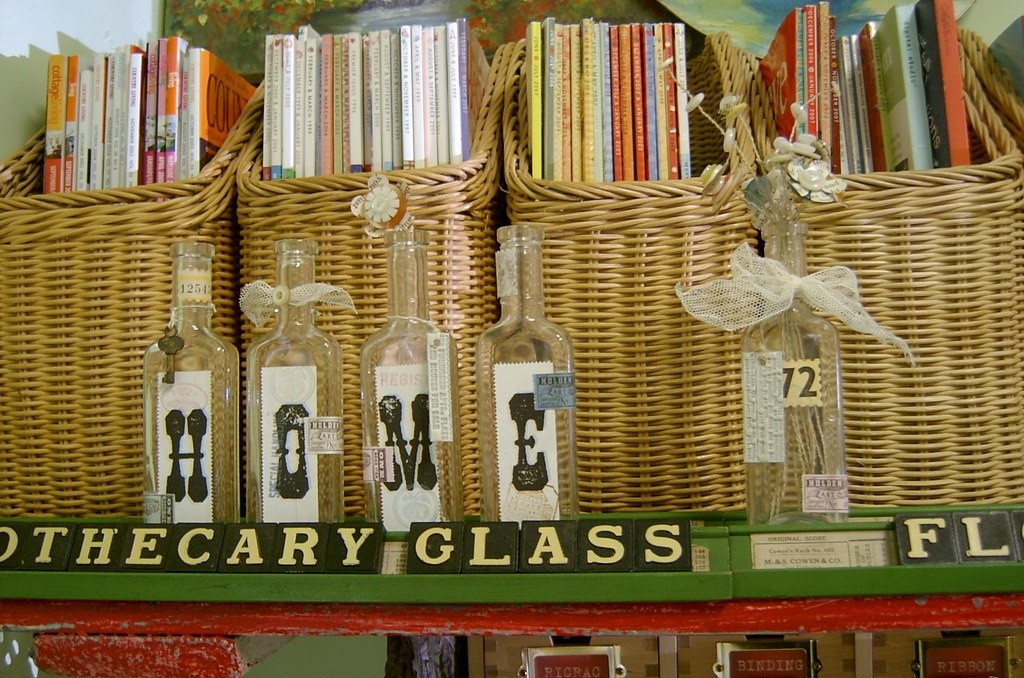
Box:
[478,224,580,520]
[143,241,240,527]
[360,231,459,532]
[246,238,345,526]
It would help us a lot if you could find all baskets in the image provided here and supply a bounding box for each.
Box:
[752,26,1023,506]
[237,40,511,521]
[1,76,263,525]
[503,33,762,513]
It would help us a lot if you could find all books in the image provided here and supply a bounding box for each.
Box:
[41,1,971,197]
